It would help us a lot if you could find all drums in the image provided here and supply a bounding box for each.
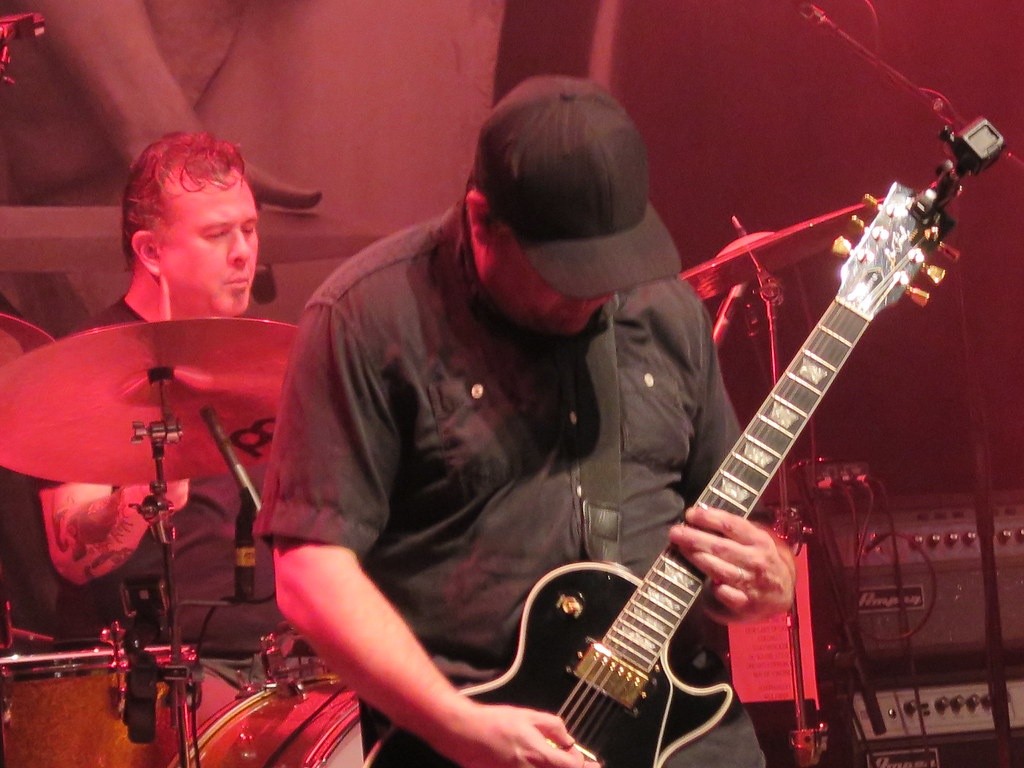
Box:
[0,644,197,768]
[166,671,365,768]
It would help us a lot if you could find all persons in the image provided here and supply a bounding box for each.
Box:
[251,74,799,768]
[36,131,291,657]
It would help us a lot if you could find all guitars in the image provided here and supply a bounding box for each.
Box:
[363,181,962,768]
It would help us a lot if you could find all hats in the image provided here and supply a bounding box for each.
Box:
[474,77,682,298]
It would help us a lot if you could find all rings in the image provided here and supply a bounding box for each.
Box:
[735,569,744,586]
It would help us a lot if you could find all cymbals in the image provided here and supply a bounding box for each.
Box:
[0,316,300,486]
[0,313,57,368]
